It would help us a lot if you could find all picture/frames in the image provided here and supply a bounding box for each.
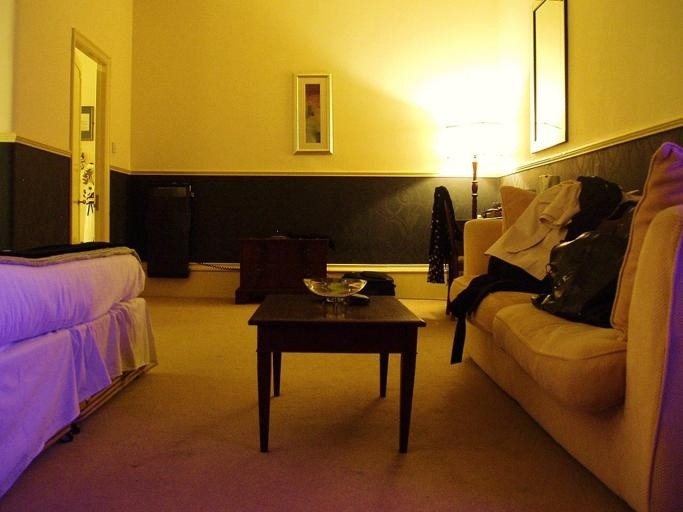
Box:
[81,106,94,141]
[530,1,567,153]
[293,74,333,155]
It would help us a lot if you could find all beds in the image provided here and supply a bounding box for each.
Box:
[0,242,159,496]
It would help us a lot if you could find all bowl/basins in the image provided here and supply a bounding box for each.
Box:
[304,278,367,298]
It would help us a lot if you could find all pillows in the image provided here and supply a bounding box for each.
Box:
[608,142,683,341]
[501,185,538,234]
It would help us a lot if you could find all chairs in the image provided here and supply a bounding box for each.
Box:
[434,186,464,321]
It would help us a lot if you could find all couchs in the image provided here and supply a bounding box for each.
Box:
[449,206,683,511]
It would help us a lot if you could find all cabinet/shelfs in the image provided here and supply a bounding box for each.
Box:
[235,239,337,305]
[149,198,191,278]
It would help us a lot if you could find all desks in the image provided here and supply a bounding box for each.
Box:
[249,292,427,455]
[453,220,474,241]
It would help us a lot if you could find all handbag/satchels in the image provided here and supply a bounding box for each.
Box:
[540,212,626,321]
